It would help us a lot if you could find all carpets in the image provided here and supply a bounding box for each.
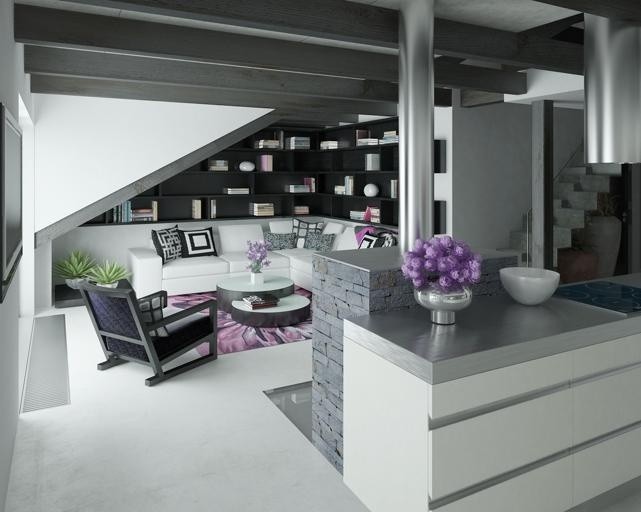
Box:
[157,279,311,366]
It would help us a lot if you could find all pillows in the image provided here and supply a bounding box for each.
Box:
[289,217,324,250]
[176,226,218,258]
[352,226,398,250]
[263,231,297,250]
[151,224,182,264]
[304,230,335,254]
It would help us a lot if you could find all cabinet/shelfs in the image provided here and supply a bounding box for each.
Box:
[87,118,401,229]
[342,273,640,512]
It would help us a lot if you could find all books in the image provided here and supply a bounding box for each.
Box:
[206,158,228,172]
[191,199,202,220]
[289,174,316,216]
[391,179,399,199]
[363,153,380,171]
[104,198,158,222]
[247,201,274,217]
[254,134,339,151]
[333,175,354,195]
[353,128,398,146]
[259,154,273,172]
[211,199,218,219]
[348,202,380,224]
[242,293,281,311]
[222,187,249,195]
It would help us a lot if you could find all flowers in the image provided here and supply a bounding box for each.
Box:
[235,239,275,273]
[396,234,482,293]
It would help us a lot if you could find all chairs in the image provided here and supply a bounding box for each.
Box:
[73,278,222,389]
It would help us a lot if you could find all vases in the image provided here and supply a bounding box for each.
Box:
[412,280,471,327]
[243,271,265,289]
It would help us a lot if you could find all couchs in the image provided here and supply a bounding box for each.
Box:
[122,220,290,299]
[264,218,398,294]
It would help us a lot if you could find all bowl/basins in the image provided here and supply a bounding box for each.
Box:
[499,266,561,306]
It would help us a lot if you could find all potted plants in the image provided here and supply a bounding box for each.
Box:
[85,259,130,291]
[54,249,92,295]
